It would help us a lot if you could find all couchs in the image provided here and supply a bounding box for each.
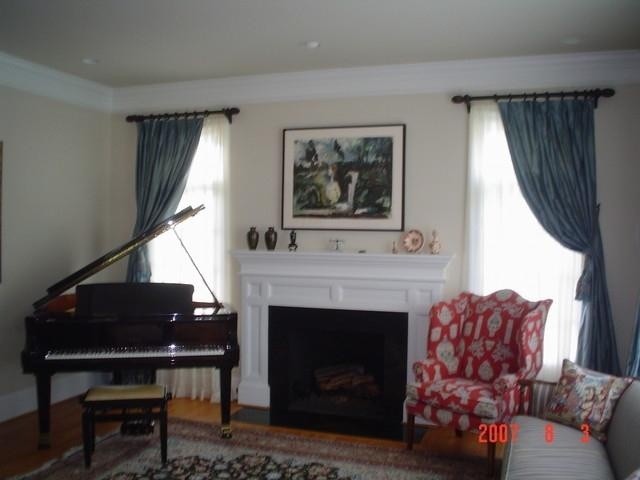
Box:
[499,378,639,479]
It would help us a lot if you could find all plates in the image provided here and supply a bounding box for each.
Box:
[403,229,424,255]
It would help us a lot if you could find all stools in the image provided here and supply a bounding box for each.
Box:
[81,384,172,469]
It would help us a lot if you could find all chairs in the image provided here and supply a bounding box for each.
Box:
[405,289,554,479]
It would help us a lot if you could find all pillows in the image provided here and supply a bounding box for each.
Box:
[539,358,635,442]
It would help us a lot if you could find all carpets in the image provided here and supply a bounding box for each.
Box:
[3,416,502,479]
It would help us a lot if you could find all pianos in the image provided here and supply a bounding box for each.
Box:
[20,204,239,450]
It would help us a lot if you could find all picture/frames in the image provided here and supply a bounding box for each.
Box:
[281,123,406,232]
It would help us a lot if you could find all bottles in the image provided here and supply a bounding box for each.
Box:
[429,231,441,255]
[248,226,258,250]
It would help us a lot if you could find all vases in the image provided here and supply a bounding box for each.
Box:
[247,227,259,250]
